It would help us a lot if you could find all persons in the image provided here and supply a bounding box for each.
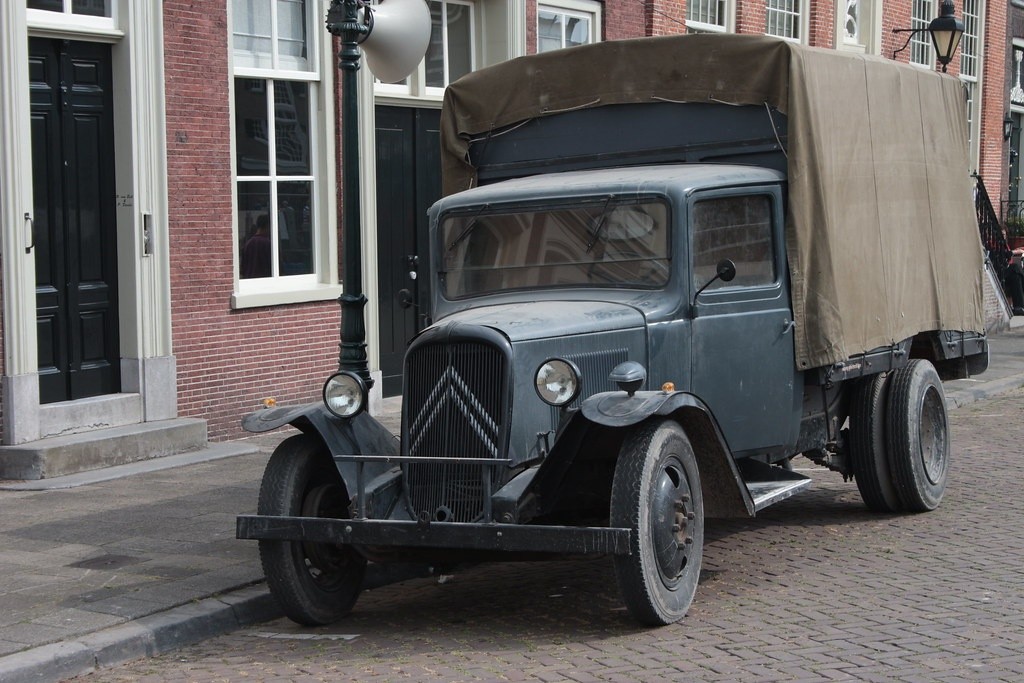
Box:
[987,225,1024,316]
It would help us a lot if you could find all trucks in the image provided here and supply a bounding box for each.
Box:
[229,32,994,623]
